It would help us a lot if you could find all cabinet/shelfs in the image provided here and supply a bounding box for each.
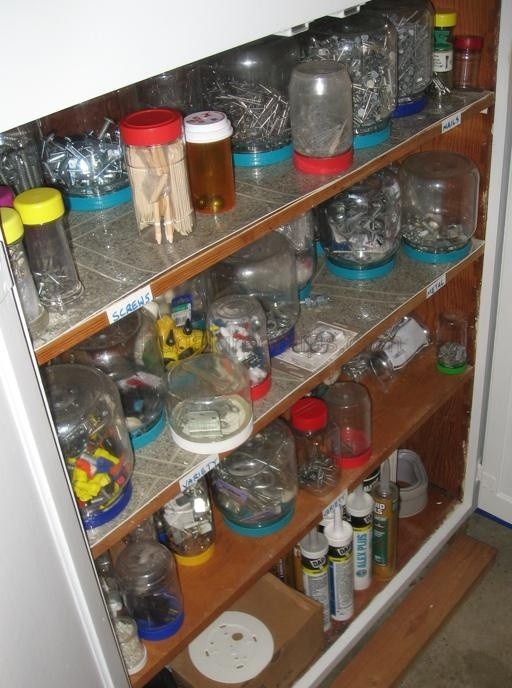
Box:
[0,0,512,688]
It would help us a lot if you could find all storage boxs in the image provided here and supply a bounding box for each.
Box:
[165,572,323,688]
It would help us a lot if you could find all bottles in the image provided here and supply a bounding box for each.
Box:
[0,2,483,679]
